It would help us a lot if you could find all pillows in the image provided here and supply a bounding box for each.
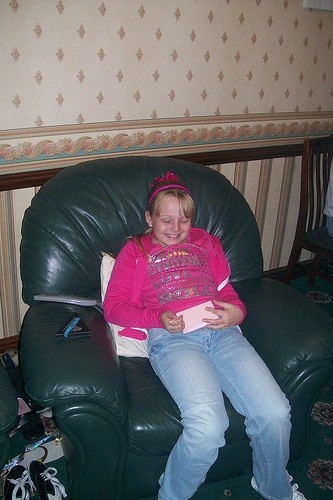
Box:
[100,250,147,357]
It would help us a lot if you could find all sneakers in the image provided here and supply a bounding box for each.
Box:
[27,459,67,500]
[3,464,33,500]
[251,475,307,500]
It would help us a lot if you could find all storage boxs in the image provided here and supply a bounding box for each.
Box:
[19,439,71,499]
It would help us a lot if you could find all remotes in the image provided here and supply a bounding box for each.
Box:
[33,293,98,306]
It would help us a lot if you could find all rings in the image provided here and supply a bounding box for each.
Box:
[170,319,173,326]
[171,327,174,331]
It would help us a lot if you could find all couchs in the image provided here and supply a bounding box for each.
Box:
[19,155,333,500]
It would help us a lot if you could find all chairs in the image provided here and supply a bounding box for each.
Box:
[283,134,333,286]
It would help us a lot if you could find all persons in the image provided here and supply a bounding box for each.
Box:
[323,155,333,240]
[102,171,310,500]
